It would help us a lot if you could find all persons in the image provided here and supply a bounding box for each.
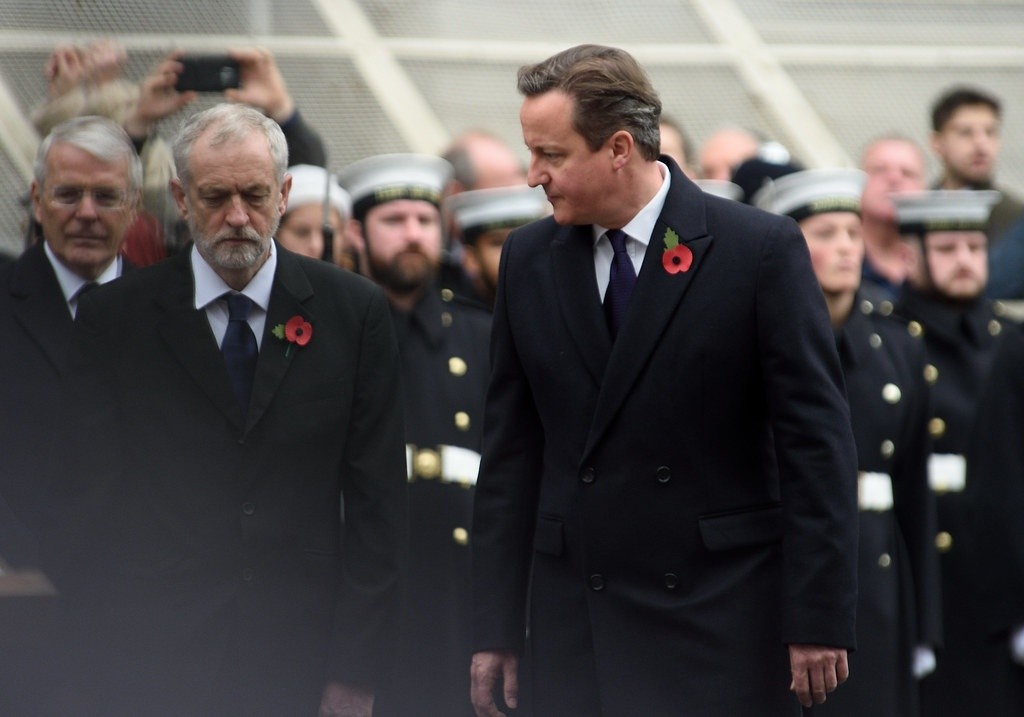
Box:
[0,114,144,717]
[469,43,860,717]
[20,39,1024,717]
[76,101,406,717]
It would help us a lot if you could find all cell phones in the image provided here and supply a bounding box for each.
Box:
[172,53,244,91]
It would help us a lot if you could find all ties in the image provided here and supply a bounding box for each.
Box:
[73,281,99,328]
[602,226,639,342]
[220,293,259,424]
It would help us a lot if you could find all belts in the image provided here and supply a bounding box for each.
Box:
[926,451,969,491]
[858,471,895,513]
[404,444,480,486]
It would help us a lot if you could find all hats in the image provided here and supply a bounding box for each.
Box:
[888,190,1005,232]
[694,178,744,202]
[282,162,351,221]
[444,184,553,246]
[749,167,868,223]
[338,153,455,217]
